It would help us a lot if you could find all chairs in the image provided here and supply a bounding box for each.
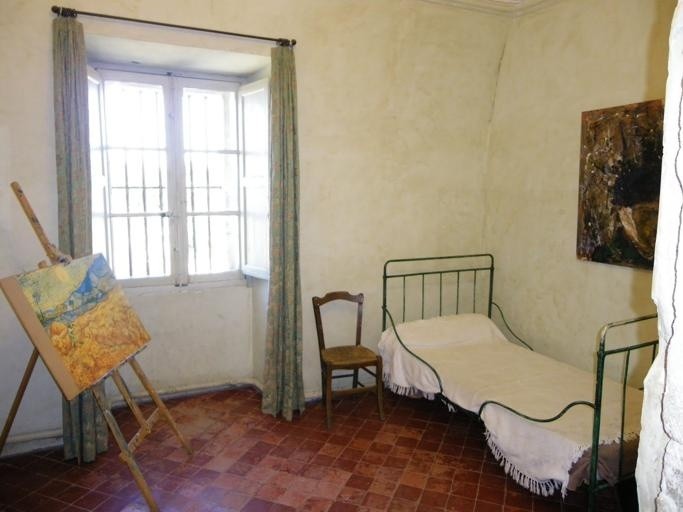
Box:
[313,291,385,429]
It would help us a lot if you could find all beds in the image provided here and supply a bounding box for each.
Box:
[377,253,657,510]
